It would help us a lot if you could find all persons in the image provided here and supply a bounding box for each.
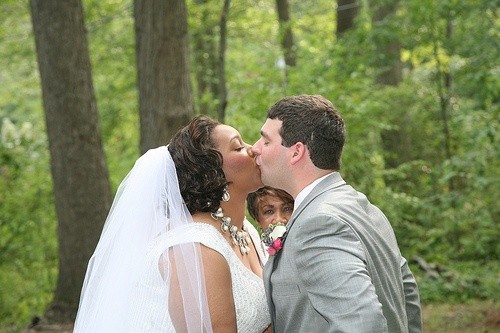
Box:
[252,95,423,332]
[73,112,273,332]
[246,186,294,232]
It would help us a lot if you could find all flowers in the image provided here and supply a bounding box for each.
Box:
[260,221,288,256]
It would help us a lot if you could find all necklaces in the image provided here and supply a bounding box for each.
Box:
[210,207,252,255]
